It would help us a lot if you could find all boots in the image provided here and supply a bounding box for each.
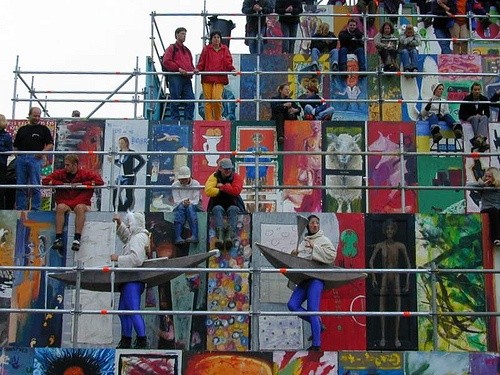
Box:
[358,68,367,80]
[226,226,234,250]
[173,223,185,245]
[215,225,224,250]
[116,336,132,349]
[454,124,463,138]
[133,335,147,349]
[186,222,200,244]
[431,126,443,143]
[340,67,349,79]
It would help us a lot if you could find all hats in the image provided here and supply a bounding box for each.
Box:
[219,158,234,169]
[178,167,191,179]
[430,81,443,95]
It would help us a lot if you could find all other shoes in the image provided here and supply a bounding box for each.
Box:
[384,64,396,71]
[470,136,490,152]
[305,113,316,120]
[279,137,284,144]
[311,65,317,71]
[72,240,80,251]
[306,345,321,352]
[332,64,337,71]
[307,325,325,341]
[51,239,64,249]
[493,239,500,245]
[405,68,418,78]
[324,115,330,120]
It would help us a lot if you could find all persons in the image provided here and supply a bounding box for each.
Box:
[113,212,147,350]
[374,21,400,72]
[0,113,13,210]
[420,82,464,139]
[470,167,500,245]
[171,166,202,244]
[338,18,366,72]
[204,158,248,251]
[286,214,338,351]
[242,0,500,55]
[490,90,500,122]
[42,154,104,251]
[163,27,196,121]
[458,83,491,150]
[399,24,421,78]
[193,31,237,120]
[300,86,335,122]
[271,84,302,142]
[12,107,54,212]
[310,22,339,71]
